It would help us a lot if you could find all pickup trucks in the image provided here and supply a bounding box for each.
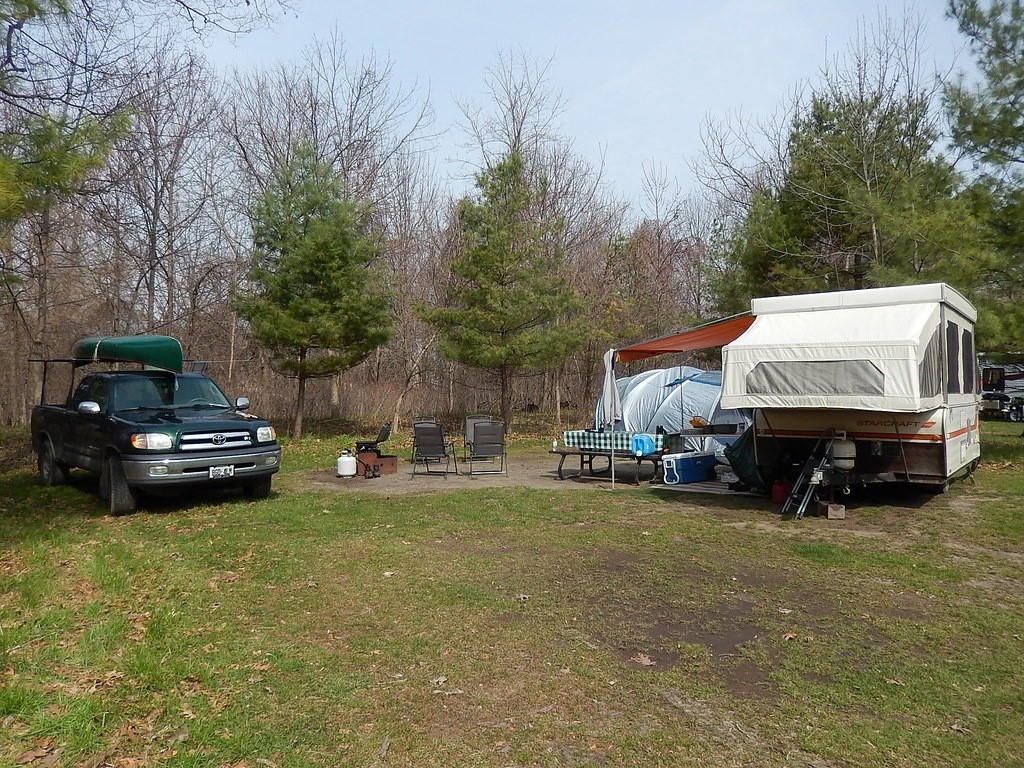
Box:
[27,334,282,517]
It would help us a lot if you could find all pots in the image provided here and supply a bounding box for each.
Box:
[355,422,392,450]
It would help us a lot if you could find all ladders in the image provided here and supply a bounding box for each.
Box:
[781,429,835,519]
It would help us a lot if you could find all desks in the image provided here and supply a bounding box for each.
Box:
[562,429,666,485]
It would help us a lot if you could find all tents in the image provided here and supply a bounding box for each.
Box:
[596,365,751,458]
[594,311,755,465]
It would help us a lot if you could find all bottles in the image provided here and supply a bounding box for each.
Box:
[551,436,558,452]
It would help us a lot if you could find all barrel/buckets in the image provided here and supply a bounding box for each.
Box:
[632,435,656,456]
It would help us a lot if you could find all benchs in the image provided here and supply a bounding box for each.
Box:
[549,450,661,486]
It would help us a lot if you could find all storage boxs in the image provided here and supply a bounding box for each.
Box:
[661,450,718,485]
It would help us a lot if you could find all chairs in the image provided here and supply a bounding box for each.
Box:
[981,367,1012,402]
[412,415,448,463]
[461,421,509,480]
[412,422,459,480]
[463,415,494,463]
